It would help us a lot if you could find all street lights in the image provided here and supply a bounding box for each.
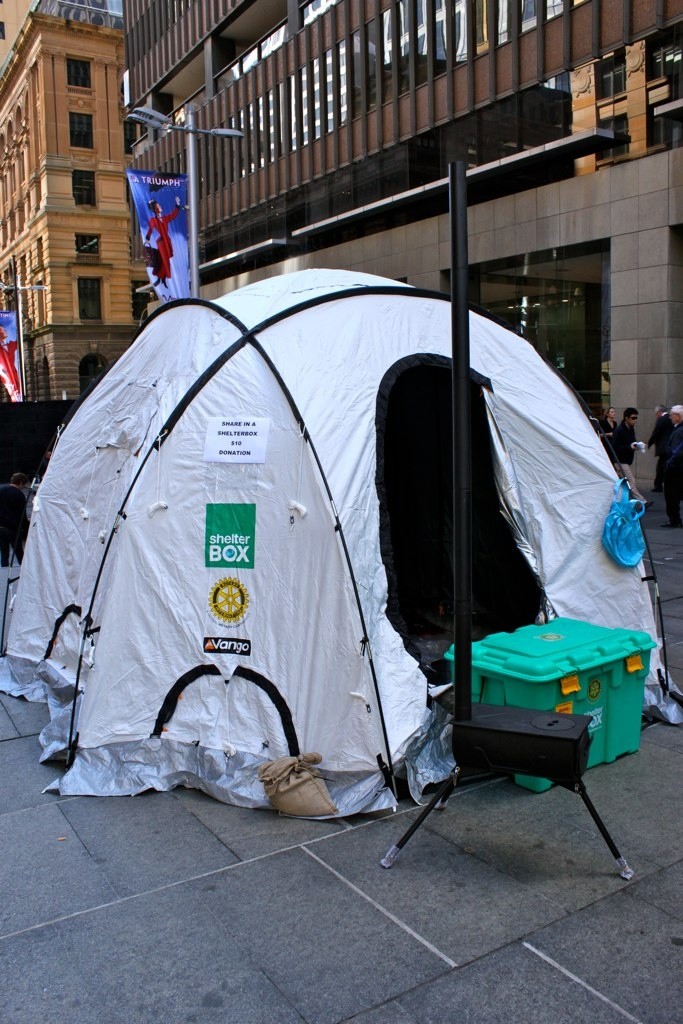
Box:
[128,108,245,298]
[0,276,45,401]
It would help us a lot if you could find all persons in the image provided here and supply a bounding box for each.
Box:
[660,405,683,528]
[37,450,51,482]
[644,404,674,492]
[596,407,617,446]
[145,196,180,288]
[610,408,654,512]
[0,324,21,403]
[0,473,30,567]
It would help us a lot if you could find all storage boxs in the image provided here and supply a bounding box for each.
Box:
[445,617,657,793]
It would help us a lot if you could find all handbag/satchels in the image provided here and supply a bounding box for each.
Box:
[600,477,647,567]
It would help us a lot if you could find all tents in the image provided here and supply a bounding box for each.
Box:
[0,268,683,820]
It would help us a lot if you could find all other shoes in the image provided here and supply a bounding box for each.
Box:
[645,500,655,508]
[661,519,683,527]
[651,488,664,492]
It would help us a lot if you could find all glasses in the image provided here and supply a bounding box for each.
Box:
[629,416,638,420]
